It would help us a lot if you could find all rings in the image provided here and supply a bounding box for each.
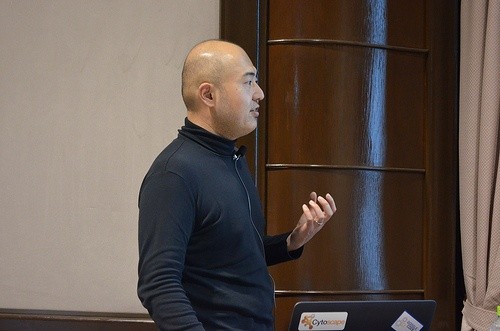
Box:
[314,219,324,225]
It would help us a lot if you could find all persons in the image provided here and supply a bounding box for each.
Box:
[136,40,338,331]
